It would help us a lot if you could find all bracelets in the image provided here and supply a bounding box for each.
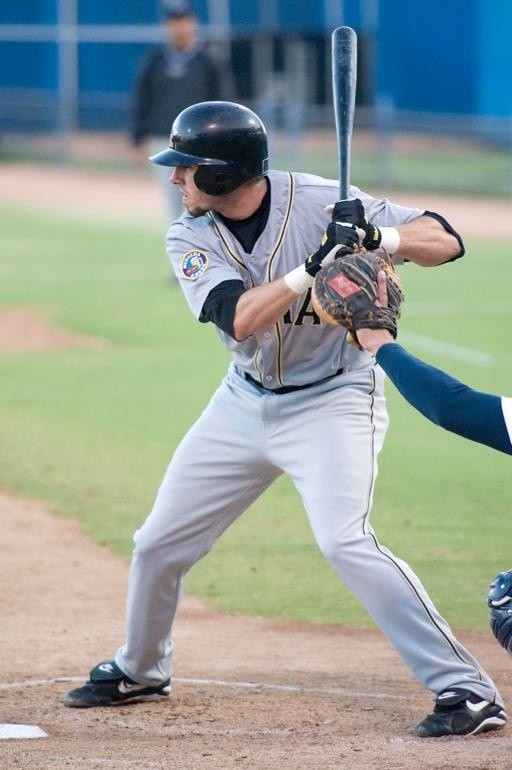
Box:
[371,224,400,255]
[284,262,315,296]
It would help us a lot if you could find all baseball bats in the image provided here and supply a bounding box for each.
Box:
[330,25,359,200]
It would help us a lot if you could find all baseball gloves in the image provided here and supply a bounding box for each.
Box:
[311,250,406,351]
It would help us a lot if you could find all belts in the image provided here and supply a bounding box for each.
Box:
[236,366,345,395]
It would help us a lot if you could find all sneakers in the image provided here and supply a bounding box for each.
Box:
[63,658,172,708]
[410,687,508,737]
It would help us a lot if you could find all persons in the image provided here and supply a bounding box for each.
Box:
[308,247,511,653]
[56,100,509,739]
[123,1,240,291]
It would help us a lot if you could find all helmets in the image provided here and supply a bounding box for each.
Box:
[148,100,269,196]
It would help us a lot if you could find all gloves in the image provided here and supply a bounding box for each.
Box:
[304,220,366,278]
[323,198,382,252]
